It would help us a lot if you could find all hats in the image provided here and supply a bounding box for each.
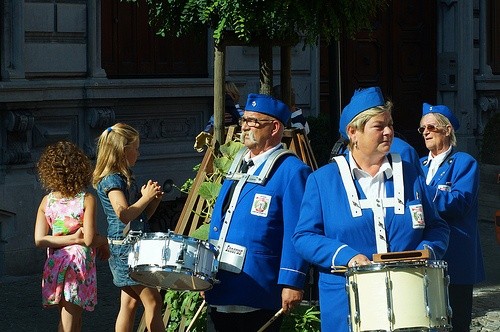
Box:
[422,103,461,131]
[245,93,291,126]
[340,86,385,139]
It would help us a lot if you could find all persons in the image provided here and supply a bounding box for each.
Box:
[91,123,166,332]
[290,88,450,332]
[203,92,313,332]
[338,136,423,170]
[271,84,311,149]
[34,140,111,332]
[223,81,244,131]
[418,103,488,332]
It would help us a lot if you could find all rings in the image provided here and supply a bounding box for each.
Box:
[355,261,359,265]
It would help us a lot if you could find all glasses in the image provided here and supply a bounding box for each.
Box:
[238,117,274,126]
[418,124,446,133]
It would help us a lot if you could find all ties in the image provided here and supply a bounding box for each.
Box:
[223,160,254,216]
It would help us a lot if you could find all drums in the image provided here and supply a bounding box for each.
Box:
[346,260,452,332]
[128,233,219,292]
